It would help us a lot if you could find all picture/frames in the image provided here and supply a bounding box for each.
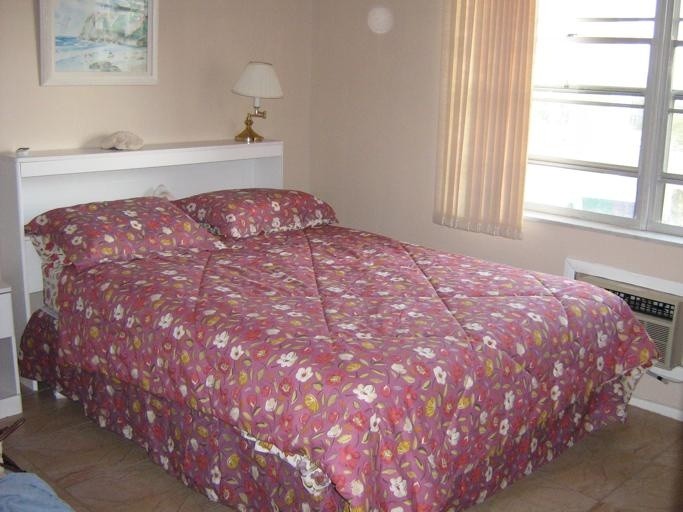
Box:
[32,0,163,88]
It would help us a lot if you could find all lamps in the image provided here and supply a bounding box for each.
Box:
[228,61,286,144]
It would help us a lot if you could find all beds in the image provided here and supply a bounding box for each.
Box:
[14,181,652,512]
